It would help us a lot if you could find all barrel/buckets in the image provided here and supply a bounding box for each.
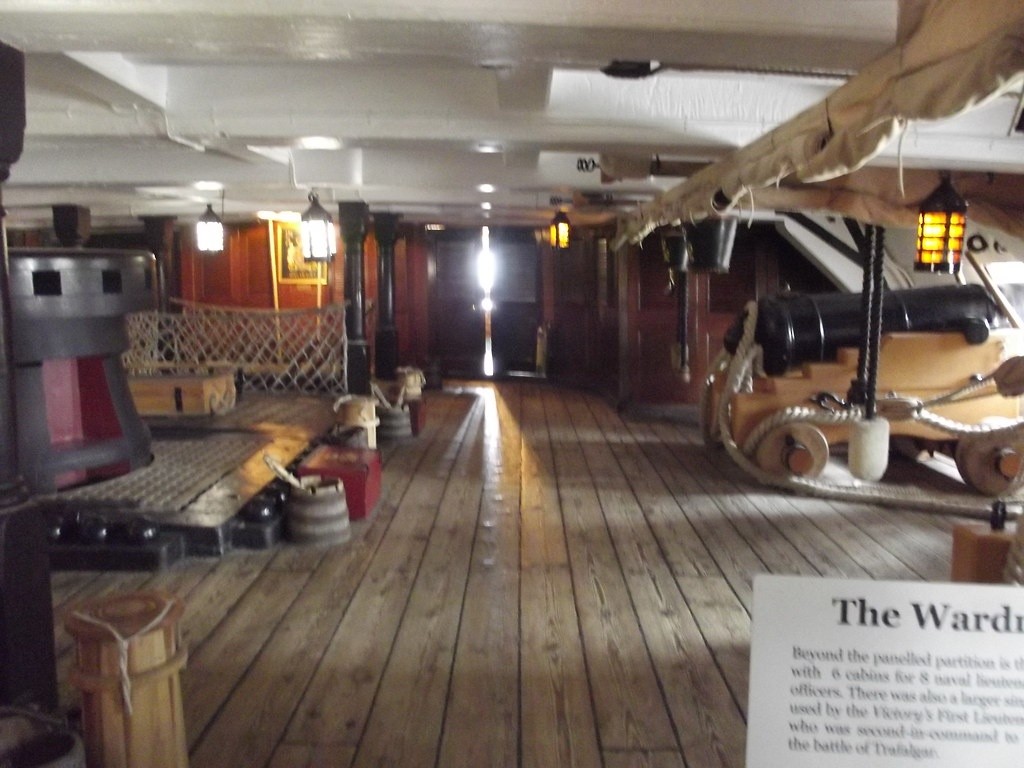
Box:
[378,405,412,436]
[289,474,350,542]
[0,714,86,768]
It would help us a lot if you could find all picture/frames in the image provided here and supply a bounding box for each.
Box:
[277,220,329,286]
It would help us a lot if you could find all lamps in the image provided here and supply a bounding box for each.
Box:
[196,204,225,252]
[909,168,969,275]
[547,210,575,251]
[299,191,339,262]
